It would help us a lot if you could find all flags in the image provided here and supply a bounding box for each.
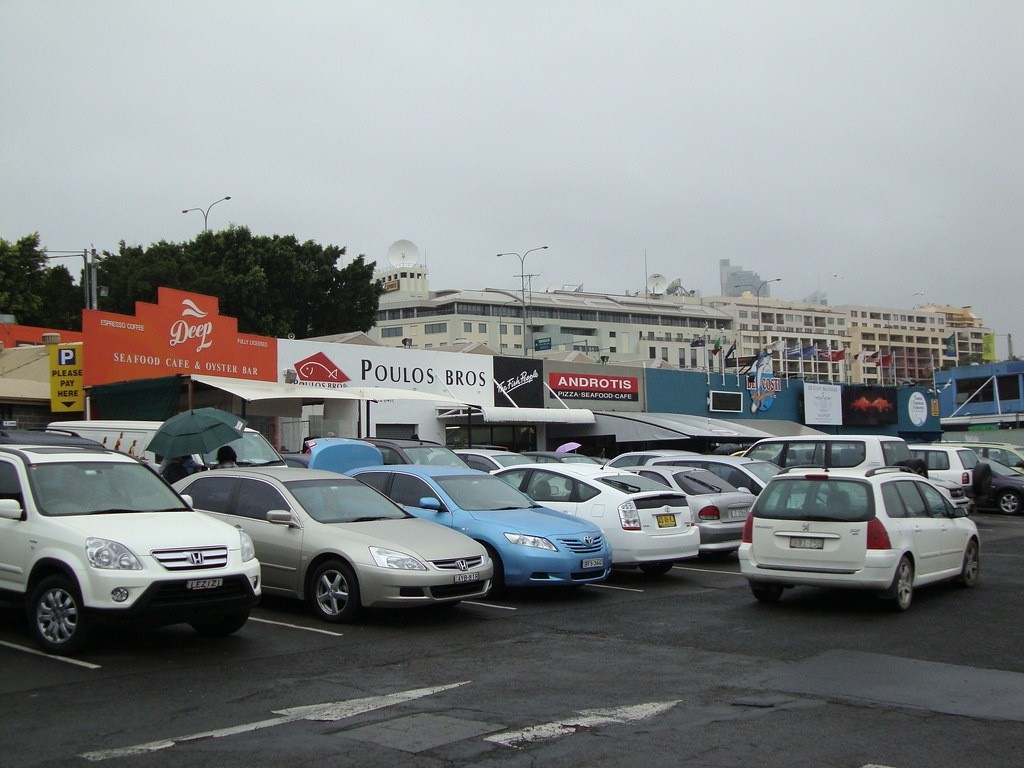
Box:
[712,336,723,356]
[924,355,934,378]
[725,337,738,360]
[946,331,958,357]
[982,333,996,360]
[762,340,894,366]
[690,334,707,347]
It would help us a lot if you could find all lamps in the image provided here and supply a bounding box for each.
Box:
[401,337,413,349]
[600,356,609,364]
[747,373,755,383]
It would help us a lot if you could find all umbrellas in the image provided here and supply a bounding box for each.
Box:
[144,408,249,466]
[715,444,742,455]
[555,442,582,452]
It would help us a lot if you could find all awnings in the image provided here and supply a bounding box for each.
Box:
[546,411,832,442]
[435,405,598,423]
[191,374,482,411]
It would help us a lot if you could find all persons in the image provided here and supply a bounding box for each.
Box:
[158,445,239,485]
[102,432,138,455]
[408,433,430,465]
[302,432,336,454]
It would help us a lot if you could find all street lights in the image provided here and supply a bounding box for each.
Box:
[182,196,232,235]
[496,244,549,356]
[733,278,782,372]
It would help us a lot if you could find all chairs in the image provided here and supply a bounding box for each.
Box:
[826,491,851,517]
[563,478,580,501]
[531,480,552,500]
[831,453,841,466]
[841,448,857,466]
[992,451,1010,466]
[803,450,816,465]
[785,449,798,467]
[936,457,943,469]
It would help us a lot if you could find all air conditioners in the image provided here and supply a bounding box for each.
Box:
[284,369,297,384]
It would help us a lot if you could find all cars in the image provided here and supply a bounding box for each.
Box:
[0,419,1024,658]
